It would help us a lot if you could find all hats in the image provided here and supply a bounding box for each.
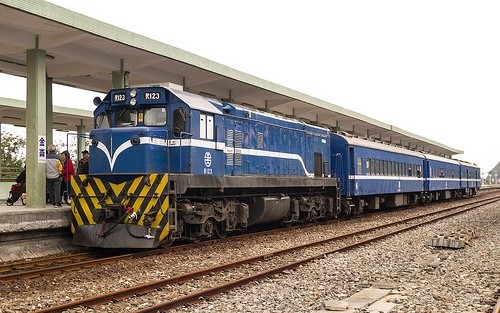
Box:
[81,151,90,154]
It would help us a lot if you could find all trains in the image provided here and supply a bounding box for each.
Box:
[70,82,482,248]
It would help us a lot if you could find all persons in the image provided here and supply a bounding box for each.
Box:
[6,165,26,204]
[46,144,63,208]
[46,150,75,206]
[76,150,89,174]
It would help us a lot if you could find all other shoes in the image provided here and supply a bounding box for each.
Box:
[7,198,14,205]
[55,202,62,208]
